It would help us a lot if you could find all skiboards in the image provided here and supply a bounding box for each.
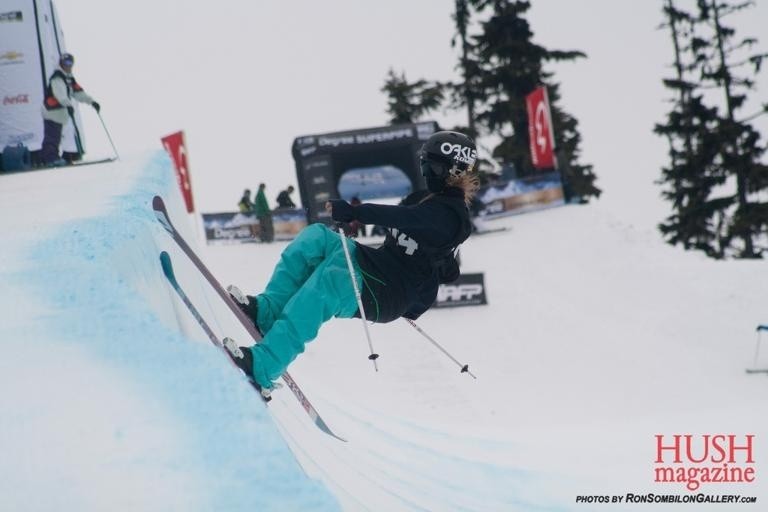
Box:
[31,159,115,169]
[152,196,345,442]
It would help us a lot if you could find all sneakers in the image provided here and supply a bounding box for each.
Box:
[233,295,257,327]
[232,346,253,378]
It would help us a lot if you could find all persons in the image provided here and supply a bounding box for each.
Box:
[349,196,368,238]
[225,129,481,404]
[238,187,256,215]
[277,186,296,207]
[41,52,103,168]
[254,183,276,244]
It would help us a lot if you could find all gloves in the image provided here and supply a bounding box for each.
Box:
[92,101,100,113]
[67,106,74,116]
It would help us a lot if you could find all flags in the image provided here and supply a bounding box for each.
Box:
[161,131,195,213]
[523,86,554,169]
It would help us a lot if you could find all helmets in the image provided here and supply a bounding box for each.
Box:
[60,53,74,67]
[420,131,477,192]
